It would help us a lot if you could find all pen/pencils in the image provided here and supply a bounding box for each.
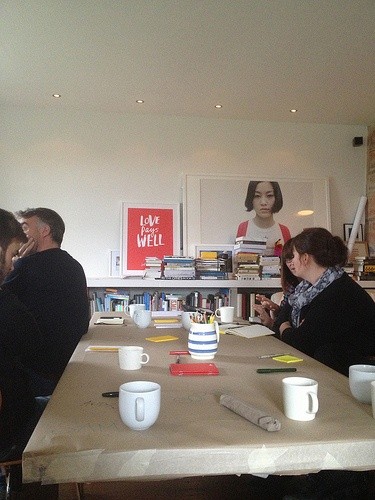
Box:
[101,392,119,397]
[257,367,298,374]
[194,309,216,323]
[256,353,293,358]
[100,316,122,319]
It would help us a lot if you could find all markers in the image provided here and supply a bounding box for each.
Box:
[170,351,189,354]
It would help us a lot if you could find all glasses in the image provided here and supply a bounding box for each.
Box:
[286,257,292,262]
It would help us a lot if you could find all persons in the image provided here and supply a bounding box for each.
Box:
[237,181,292,257]
[280,228,375,374]
[2,207,91,395]
[252,237,313,339]
[0,209,60,500]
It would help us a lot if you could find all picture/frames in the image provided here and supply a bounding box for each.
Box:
[108,248,120,278]
[343,223,363,242]
[106,294,130,312]
[183,173,332,259]
[344,241,369,261]
[121,200,181,277]
[194,244,235,278]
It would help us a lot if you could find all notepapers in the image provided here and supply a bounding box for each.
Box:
[275,354,304,363]
[144,335,178,343]
[154,317,179,323]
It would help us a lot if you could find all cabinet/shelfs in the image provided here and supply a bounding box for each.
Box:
[87,278,375,317]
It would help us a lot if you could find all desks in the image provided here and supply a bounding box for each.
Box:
[20,311,375,500]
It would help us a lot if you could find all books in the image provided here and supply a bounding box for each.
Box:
[84,235,375,323]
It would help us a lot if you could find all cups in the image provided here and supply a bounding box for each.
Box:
[187,321,220,360]
[181,311,202,332]
[134,309,152,329]
[215,307,235,323]
[123,303,146,320]
[348,364,375,403]
[118,381,161,431]
[281,377,319,422]
[370,380,375,419]
[118,346,150,371]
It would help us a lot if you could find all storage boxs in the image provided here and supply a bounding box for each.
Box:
[166,297,178,311]
